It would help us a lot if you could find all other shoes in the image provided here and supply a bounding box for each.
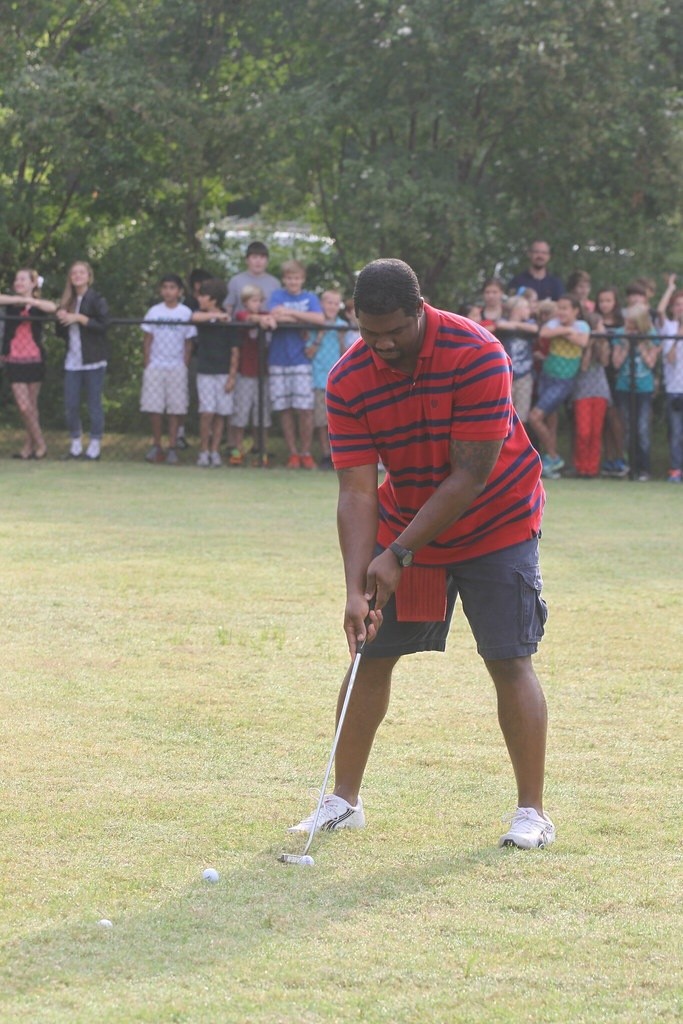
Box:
[541,452,683,483]
[176,436,188,449]
[165,447,179,463]
[211,451,222,466]
[225,445,234,457]
[228,447,243,465]
[287,454,300,469]
[320,456,331,471]
[146,444,163,461]
[197,450,210,467]
[86,437,101,459]
[21,449,34,460]
[252,447,267,466]
[250,447,271,456]
[300,453,317,471]
[70,433,83,456]
[34,450,47,459]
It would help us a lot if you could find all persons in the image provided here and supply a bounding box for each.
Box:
[287,258,557,852]
[55,260,110,460]
[0,269,58,459]
[139,274,196,463]
[183,242,388,470]
[458,239,683,482]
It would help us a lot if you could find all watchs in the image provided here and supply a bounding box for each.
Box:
[389,542,413,567]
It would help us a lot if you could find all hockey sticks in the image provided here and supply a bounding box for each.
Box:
[277,589,378,865]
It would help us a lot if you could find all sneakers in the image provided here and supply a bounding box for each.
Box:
[499,807,555,849]
[287,795,365,830]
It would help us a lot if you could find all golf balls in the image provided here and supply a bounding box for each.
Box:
[298,856,314,865]
[202,868,219,882]
[98,919,113,929]
